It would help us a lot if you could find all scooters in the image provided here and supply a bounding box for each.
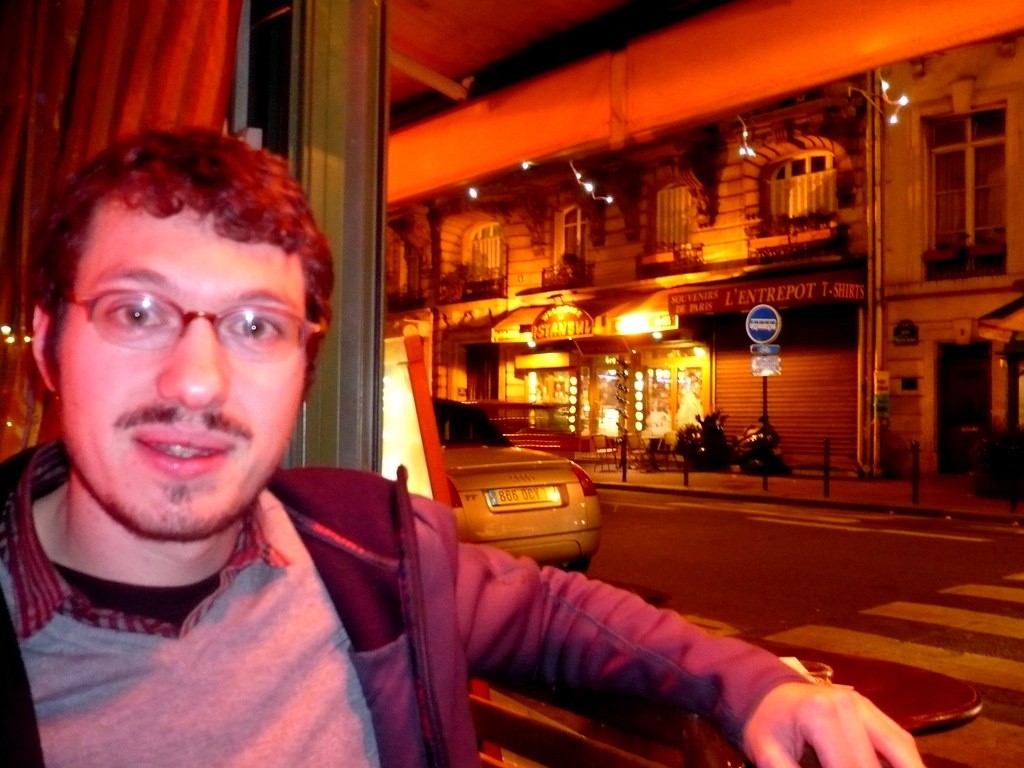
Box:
[695,410,779,469]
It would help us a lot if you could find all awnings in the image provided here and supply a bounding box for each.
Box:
[447,270,870,344]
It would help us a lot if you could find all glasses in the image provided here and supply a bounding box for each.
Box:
[49,290,321,362]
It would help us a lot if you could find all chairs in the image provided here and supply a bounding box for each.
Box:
[656,431,681,472]
[591,435,617,473]
[626,433,647,469]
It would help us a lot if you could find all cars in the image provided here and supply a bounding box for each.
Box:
[426,396,601,570]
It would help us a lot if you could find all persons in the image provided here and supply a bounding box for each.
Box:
[0,125,930,768]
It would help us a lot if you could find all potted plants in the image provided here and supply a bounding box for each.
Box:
[673,425,707,472]
